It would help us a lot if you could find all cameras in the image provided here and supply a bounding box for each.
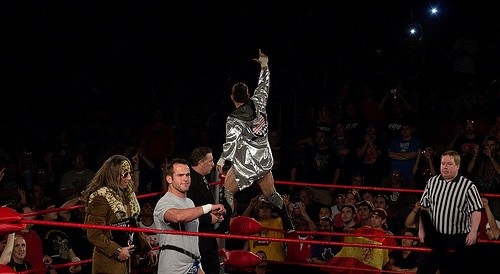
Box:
[293,202,300,208]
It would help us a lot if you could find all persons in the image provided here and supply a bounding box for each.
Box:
[418,151,484,274]
[79,155,158,274]
[153,158,226,274]
[216,49,295,235]
[0,83,500,274]
[187,147,220,274]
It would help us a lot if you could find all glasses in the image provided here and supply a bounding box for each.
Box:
[120,171,132,178]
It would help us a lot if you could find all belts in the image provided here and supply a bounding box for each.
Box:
[160,244,200,260]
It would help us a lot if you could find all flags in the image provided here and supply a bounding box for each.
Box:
[326,225,398,274]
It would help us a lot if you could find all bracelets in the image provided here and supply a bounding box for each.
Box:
[412,209,417,213]
[133,169,140,172]
[201,203,212,214]
[114,246,122,256]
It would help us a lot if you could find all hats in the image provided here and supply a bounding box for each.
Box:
[373,208,387,219]
